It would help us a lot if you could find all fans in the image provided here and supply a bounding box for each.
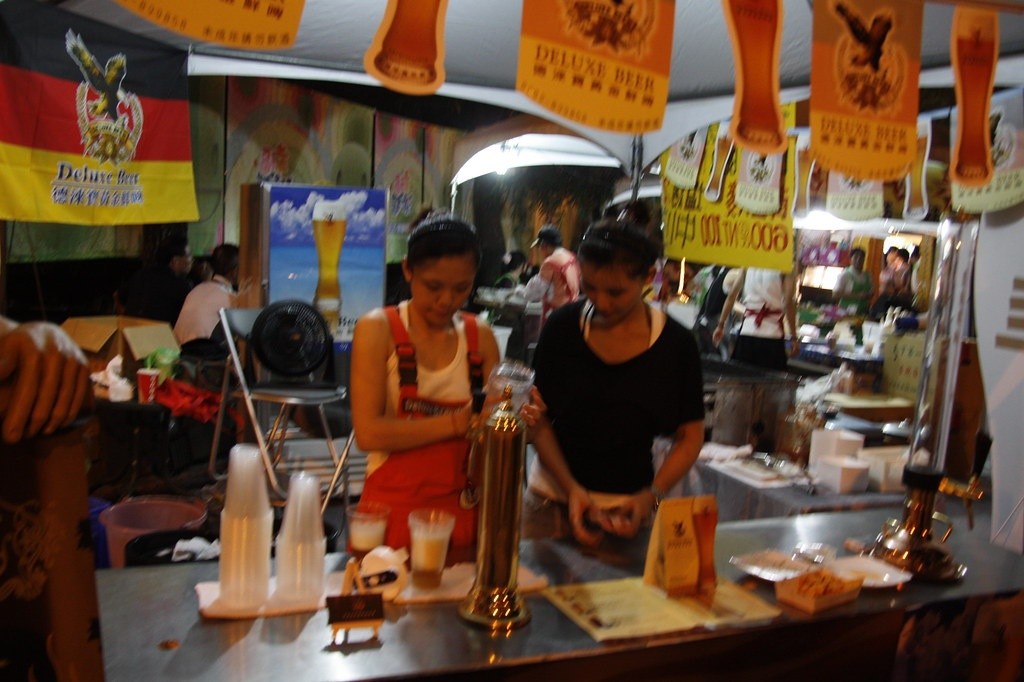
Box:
[250,300,336,391]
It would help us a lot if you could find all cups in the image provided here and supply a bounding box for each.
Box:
[706,121,732,201]
[827,333,836,350]
[863,337,874,353]
[408,509,455,586]
[137,368,164,401]
[721,0,790,154]
[950,4,997,185]
[220,445,324,608]
[901,118,932,221]
[363,0,451,95]
[789,133,817,218]
[345,504,389,558]
[311,217,346,304]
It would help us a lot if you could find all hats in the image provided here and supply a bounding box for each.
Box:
[531,226,562,249]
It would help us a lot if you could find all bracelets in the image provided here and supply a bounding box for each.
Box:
[646,485,663,511]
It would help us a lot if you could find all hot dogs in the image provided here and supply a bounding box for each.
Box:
[586,492,636,535]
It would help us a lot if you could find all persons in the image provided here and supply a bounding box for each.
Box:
[0,319,92,444]
[348,205,547,551]
[644,243,932,449]
[489,226,581,356]
[129,233,243,363]
[523,217,706,544]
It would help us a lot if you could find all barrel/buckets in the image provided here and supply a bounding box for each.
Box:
[100,499,206,569]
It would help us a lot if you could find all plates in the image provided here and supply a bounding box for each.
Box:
[730,547,915,589]
[809,426,873,496]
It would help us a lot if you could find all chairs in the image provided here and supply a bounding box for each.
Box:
[208,309,347,499]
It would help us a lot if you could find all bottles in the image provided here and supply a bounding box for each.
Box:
[469,363,536,491]
